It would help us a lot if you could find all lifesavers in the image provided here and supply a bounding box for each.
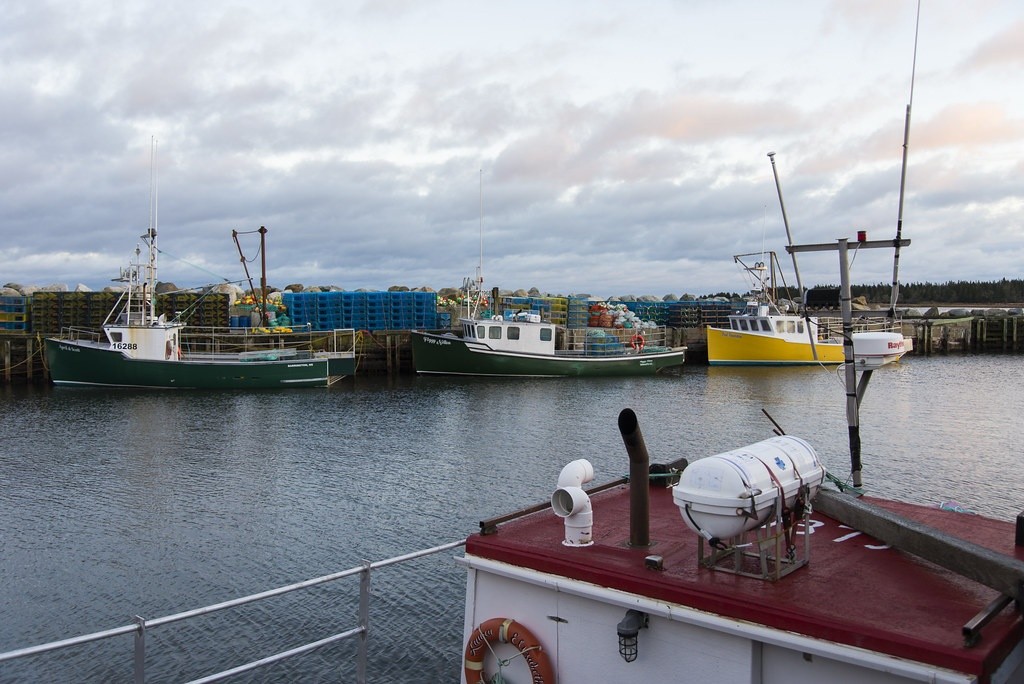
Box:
[465,618,556,683]
[630,334,644,350]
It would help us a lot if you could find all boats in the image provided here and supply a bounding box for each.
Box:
[702,151,850,366]
[39,133,359,390]
[415,1,1024,684]
[408,167,690,375]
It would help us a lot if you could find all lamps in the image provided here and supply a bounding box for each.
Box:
[617,610,648,663]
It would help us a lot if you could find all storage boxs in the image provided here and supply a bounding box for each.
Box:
[283,291,451,330]
[0,291,230,333]
[481,296,767,355]
